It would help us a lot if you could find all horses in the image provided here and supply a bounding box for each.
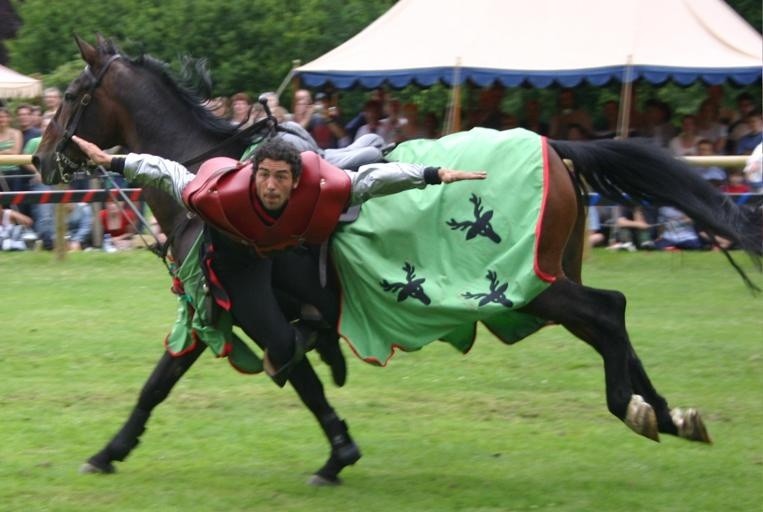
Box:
[30,28,762,487]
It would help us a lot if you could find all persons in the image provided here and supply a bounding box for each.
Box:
[459,86,576,141]
[0,76,159,252]
[571,83,763,251]
[203,84,432,151]
[66,131,488,467]
